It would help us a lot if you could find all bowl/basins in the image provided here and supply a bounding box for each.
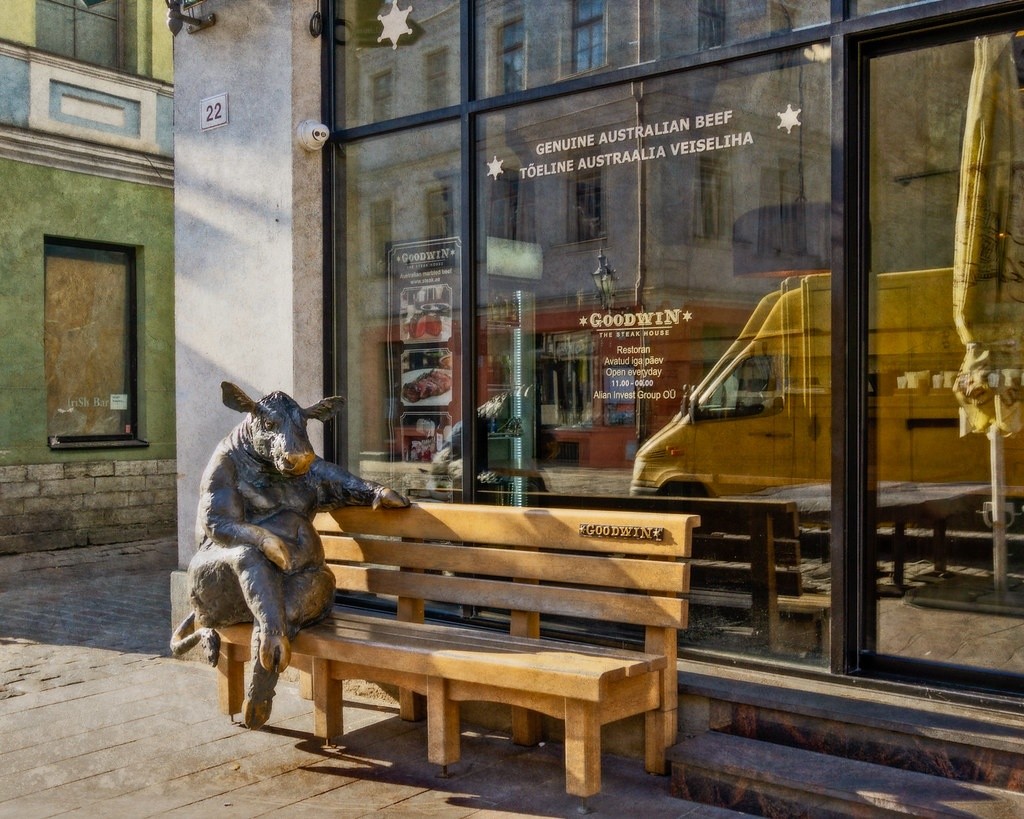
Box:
[421,304,449,316]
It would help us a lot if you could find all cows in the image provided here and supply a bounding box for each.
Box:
[417,382,563,504]
[168,380,411,729]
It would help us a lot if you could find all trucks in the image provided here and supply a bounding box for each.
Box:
[626,265,1024,529]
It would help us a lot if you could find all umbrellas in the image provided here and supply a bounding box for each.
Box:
[953,34,1022,605]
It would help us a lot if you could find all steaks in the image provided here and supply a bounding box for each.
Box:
[402,369,451,403]
[409,312,442,338]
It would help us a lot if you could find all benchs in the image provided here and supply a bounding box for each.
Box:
[196,506,700,798]
[406,485,830,660]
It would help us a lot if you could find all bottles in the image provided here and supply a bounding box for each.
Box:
[435,416,447,453]
[487,354,511,384]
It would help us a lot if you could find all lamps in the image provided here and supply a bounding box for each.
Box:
[592,251,627,314]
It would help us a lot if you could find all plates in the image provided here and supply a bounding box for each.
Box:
[400,369,452,405]
[399,316,452,344]
